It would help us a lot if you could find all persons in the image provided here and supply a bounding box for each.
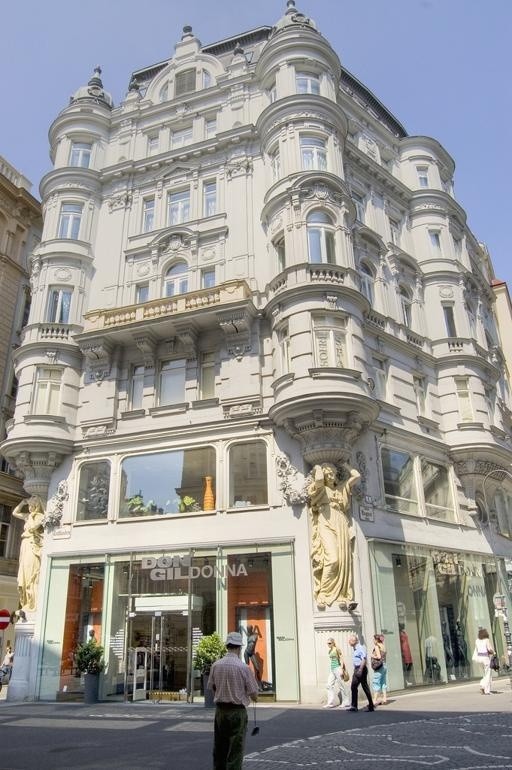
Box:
[11,495,45,617]
[322,637,350,708]
[475,629,497,695]
[370,634,387,704]
[442,621,455,674]
[346,635,374,712]
[89,630,97,642]
[241,625,264,692]
[307,459,362,609]
[207,632,260,770]
[399,622,413,686]
[0,646,15,692]
[454,621,470,680]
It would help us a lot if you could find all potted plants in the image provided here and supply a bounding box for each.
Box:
[72,636,105,703]
[192,635,229,707]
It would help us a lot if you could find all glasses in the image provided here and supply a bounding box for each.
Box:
[327,643,333,645]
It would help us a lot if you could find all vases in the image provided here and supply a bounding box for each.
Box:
[203,476,214,511]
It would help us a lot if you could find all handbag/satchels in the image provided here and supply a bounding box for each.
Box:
[370,644,383,671]
[342,668,350,682]
[490,654,500,672]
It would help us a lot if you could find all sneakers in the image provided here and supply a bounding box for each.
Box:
[363,707,374,712]
[322,703,335,708]
[346,707,359,712]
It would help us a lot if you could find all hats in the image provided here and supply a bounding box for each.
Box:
[225,632,244,647]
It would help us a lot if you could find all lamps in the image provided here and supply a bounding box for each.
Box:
[395,557,402,569]
